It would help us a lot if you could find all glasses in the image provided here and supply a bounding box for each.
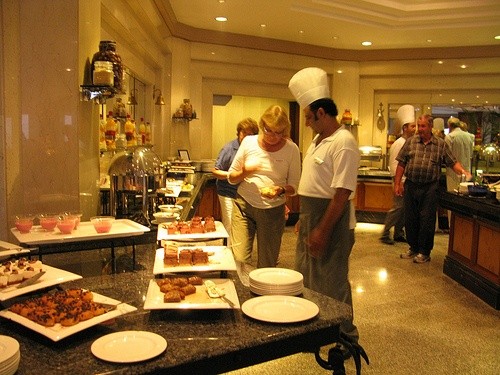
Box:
[263,127,283,137]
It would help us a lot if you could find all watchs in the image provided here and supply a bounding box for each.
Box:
[278,186,286,197]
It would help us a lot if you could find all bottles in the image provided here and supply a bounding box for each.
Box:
[138,118,145,145]
[176,99,192,117]
[124,115,134,147]
[131,119,137,146]
[342,108,352,125]
[91,62,115,85]
[146,122,151,144]
[105,111,117,149]
[117,97,127,118]
[89,40,123,90]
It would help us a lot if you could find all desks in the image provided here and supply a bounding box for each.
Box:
[351,170,393,213]
[19,232,146,277]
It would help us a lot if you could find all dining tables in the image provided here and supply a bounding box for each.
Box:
[0,263,365,375]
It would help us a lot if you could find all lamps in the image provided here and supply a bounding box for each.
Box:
[378,102,384,118]
[153,86,166,105]
[128,88,138,105]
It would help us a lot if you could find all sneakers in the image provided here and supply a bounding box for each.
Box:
[413,254,430,262]
[400,249,418,258]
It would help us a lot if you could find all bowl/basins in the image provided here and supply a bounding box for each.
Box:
[153,212,179,223]
[14,217,33,234]
[64,215,82,230]
[39,216,57,232]
[57,217,77,234]
[90,216,115,233]
[468,185,489,197]
[158,205,183,213]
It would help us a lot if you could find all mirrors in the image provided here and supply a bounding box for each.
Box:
[99,65,149,123]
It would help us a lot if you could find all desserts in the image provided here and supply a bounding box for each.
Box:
[0,256,42,289]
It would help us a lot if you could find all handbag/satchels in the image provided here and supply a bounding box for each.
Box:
[468,181,489,197]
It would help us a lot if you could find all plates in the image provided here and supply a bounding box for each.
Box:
[90,330,167,363]
[144,279,240,311]
[157,221,229,241]
[0,288,137,342]
[248,268,304,296]
[0,264,84,301]
[241,295,319,322]
[151,220,158,224]
[152,246,237,275]
[192,161,213,172]
[0,335,21,375]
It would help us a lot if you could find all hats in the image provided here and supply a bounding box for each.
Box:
[288,67,330,110]
[397,104,415,128]
[433,118,445,131]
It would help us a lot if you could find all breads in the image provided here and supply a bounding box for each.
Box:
[8,288,105,327]
[168,215,215,235]
[164,244,209,266]
[157,276,203,302]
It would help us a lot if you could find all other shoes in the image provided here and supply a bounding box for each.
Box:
[394,235,407,242]
[383,238,394,244]
[435,228,450,232]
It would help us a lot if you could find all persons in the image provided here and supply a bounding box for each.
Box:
[394,114,471,263]
[212,117,260,247]
[288,67,360,358]
[227,105,301,268]
[381,104,415,244]
[432,118,474,232]
